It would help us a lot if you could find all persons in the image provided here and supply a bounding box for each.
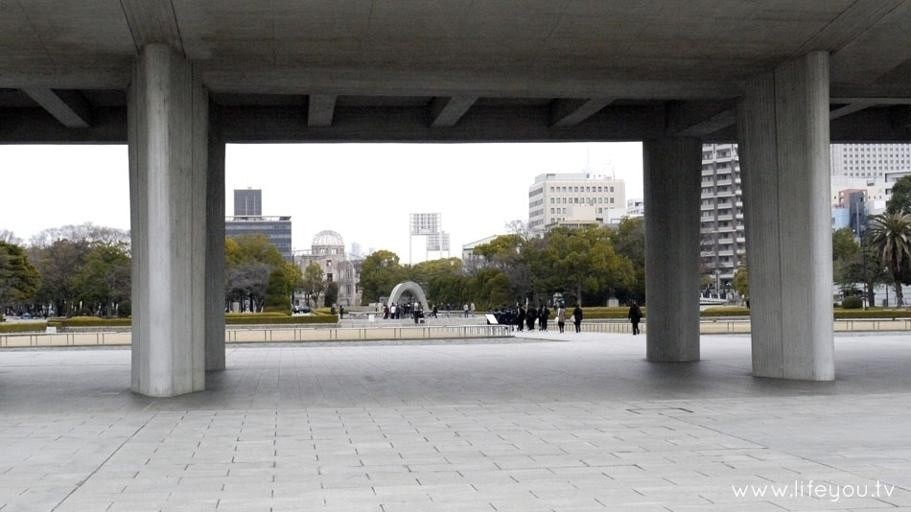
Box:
[340,305,345,318]
[487,302,584,333]
[464,303,469,317]
[470,302,476,316]
[628,303,643,335]
[383,301,439,324]
